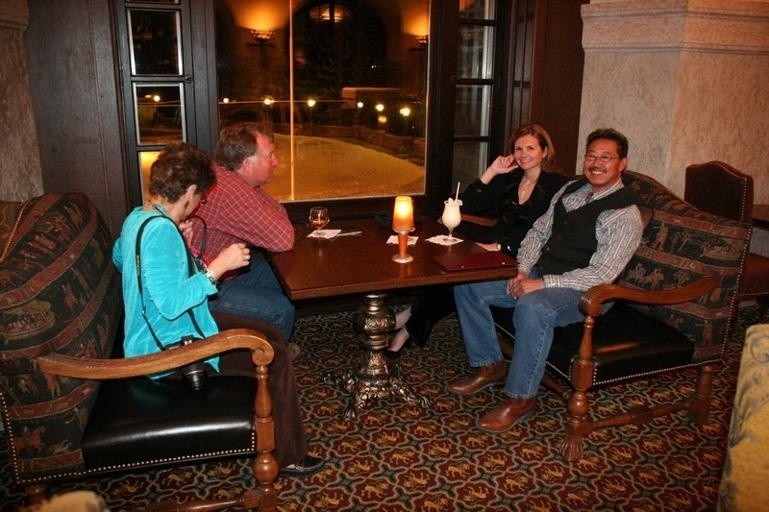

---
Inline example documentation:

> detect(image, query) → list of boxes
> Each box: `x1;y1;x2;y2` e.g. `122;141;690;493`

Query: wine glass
441;200;462;243
308;206;329;236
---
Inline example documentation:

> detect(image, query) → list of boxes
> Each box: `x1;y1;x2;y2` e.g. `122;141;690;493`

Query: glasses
584;153;622;162
198;188;208;206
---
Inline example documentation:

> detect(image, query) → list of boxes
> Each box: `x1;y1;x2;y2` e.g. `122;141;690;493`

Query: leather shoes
276;452;326;478
446;361;508;396
476;397;538;435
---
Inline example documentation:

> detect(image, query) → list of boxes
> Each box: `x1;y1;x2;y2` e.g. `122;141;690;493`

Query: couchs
476;168;754;462
2;189;291;511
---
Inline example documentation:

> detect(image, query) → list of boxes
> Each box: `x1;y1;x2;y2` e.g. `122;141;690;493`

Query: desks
260;213;520;423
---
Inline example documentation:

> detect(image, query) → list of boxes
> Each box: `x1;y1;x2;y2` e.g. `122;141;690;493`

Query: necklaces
519;178;535;202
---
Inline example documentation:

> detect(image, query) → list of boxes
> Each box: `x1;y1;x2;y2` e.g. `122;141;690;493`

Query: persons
110;142;325;479
379;123;569;361
445;126;644;435
181;115;300;364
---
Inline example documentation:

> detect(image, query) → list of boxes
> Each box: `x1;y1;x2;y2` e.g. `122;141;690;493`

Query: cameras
162;335;209;393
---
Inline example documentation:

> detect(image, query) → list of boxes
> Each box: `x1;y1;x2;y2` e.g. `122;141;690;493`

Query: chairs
680;159;769;331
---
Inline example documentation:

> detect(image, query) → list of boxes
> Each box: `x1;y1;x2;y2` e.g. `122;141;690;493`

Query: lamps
397;0;477;61
224;0;303;65
388;196;420;265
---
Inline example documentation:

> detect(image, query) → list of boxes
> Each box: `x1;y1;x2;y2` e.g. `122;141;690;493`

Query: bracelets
496;242;502;252
201;268;219;286
